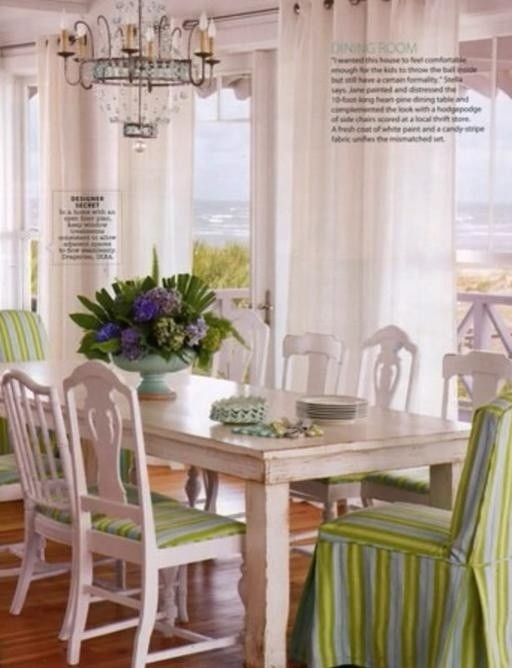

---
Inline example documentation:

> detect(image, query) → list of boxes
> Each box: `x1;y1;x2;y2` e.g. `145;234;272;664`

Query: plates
208;395;272;426
296;394;370;427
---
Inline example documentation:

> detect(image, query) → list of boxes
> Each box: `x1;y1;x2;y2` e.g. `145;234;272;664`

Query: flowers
67;245;255;369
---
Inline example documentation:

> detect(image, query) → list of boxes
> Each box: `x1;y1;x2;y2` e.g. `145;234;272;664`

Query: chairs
0;309;512;668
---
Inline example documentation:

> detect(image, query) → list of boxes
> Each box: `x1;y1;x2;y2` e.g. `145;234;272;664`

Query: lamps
57;0;221;152
